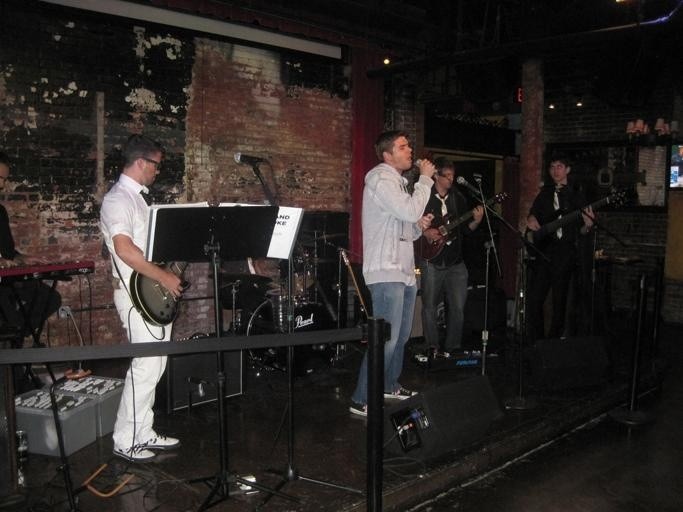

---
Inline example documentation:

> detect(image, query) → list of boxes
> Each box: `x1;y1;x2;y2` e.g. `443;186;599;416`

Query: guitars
413;192;507;260
130;261;191;327
524;190;626;257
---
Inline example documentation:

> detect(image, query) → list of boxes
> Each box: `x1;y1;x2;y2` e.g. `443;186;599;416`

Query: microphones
414;158;440;174
456;176;480;195
233;152;267;164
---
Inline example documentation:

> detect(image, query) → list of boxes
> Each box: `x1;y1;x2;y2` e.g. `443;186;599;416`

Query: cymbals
209;274;272;286
305;233;348;246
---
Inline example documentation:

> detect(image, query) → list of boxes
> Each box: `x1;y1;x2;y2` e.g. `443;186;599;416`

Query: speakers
155;349;242;413
352;375;505;471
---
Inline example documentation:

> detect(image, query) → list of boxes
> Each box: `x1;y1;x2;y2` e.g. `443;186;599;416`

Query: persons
100;134;183;464
525;159;595;348
420;164;483;357
350;134;435;417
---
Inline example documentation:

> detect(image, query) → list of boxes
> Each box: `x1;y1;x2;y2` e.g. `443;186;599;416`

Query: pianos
1;260;94;279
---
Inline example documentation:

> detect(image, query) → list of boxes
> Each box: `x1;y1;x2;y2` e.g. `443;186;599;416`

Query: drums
247;295;335;377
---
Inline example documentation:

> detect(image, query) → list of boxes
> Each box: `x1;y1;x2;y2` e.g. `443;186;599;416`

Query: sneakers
383;386;418;400
349;402;367;416
112;434;182;463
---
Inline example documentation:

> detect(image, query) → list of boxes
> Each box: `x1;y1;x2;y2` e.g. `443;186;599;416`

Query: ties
435;193;452;246
553;192;563;239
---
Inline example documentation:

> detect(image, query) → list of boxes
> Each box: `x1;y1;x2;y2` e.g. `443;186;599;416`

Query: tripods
184;235;372;512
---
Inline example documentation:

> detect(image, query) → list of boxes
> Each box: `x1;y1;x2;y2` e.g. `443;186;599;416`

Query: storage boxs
14;390;97;458
43;376;125;437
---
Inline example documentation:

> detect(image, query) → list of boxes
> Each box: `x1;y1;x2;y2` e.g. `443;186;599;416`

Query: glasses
141;156;162;170
